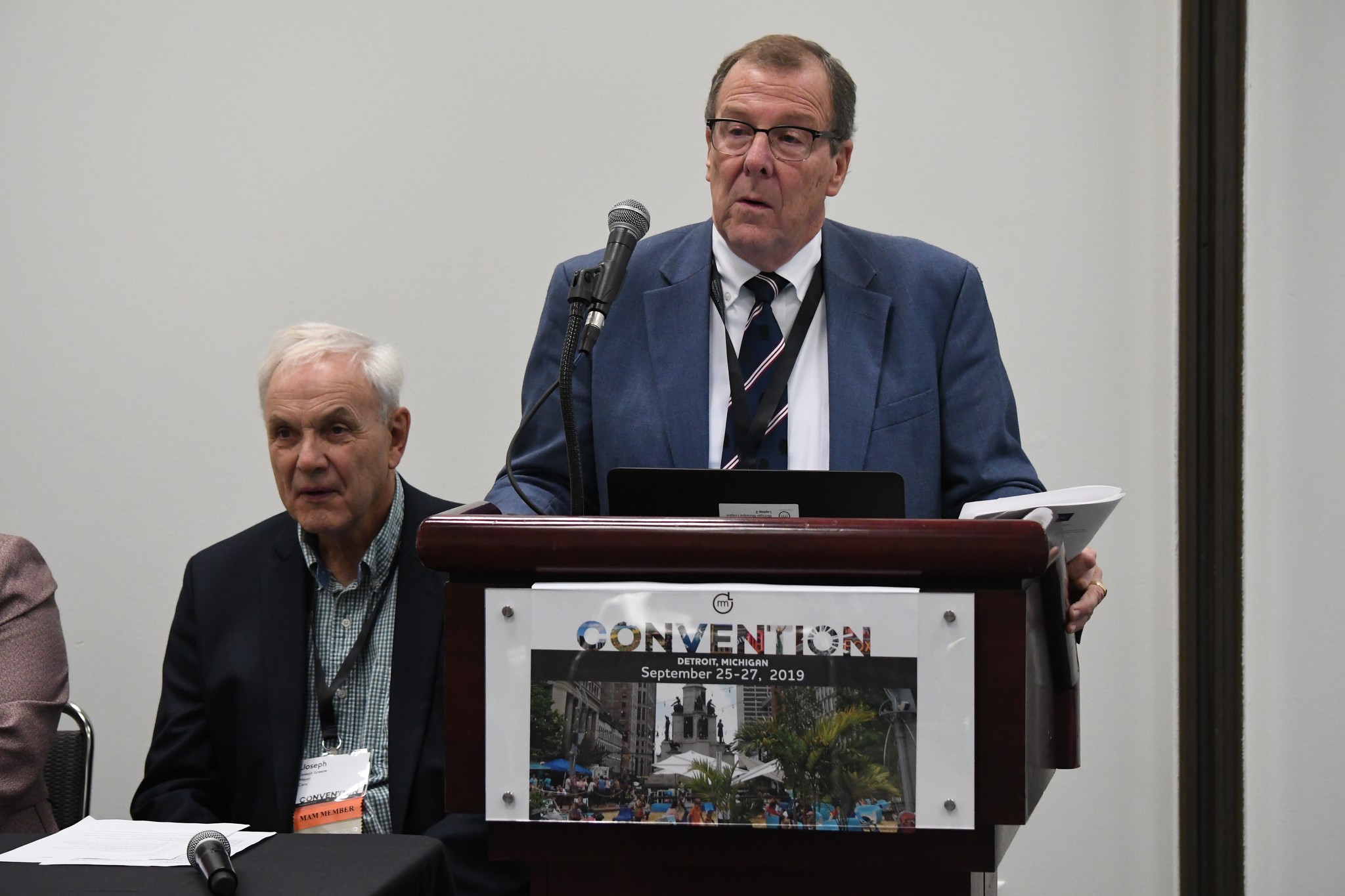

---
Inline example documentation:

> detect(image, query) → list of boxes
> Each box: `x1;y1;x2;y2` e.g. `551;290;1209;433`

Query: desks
1;834;452;896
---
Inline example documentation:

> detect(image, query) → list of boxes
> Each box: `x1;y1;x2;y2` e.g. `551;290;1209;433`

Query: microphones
186;829;238;896
577;198;651;356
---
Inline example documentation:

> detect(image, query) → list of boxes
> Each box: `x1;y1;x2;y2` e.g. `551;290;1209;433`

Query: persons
485;33;1108;644
128;319;468;834
0;534;70;836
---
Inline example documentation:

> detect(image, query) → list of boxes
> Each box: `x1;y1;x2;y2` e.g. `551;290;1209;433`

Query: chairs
43;700;95;831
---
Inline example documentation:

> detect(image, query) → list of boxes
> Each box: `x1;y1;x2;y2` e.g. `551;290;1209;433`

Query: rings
1090;581;1107;599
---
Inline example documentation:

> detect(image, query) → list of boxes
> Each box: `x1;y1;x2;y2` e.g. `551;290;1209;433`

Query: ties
719;271;791;470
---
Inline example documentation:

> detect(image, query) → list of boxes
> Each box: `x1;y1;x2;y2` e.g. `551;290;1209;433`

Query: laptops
606;466;907;519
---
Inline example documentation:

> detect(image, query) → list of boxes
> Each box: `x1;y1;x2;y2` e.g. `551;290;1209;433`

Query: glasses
706;117;836;161
